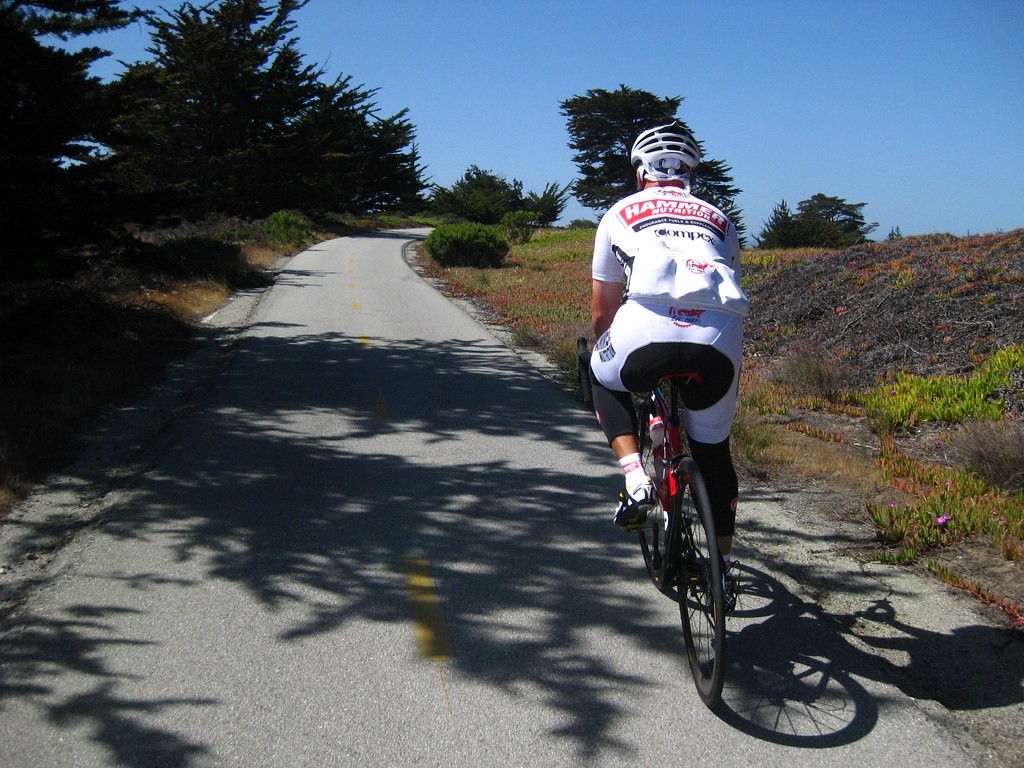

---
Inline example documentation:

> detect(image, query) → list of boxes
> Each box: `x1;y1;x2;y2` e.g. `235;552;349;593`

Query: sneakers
612;477;658;532
708;572;736;611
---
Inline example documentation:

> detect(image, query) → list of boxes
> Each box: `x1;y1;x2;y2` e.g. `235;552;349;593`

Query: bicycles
575;336;727;711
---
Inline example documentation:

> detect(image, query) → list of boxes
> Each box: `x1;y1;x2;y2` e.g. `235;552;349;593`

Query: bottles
649;415;664;450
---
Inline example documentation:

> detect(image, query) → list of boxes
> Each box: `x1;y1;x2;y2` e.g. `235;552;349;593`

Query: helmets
631;120;701;168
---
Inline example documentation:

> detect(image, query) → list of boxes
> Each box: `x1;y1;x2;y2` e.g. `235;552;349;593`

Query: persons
590;121;751;609
